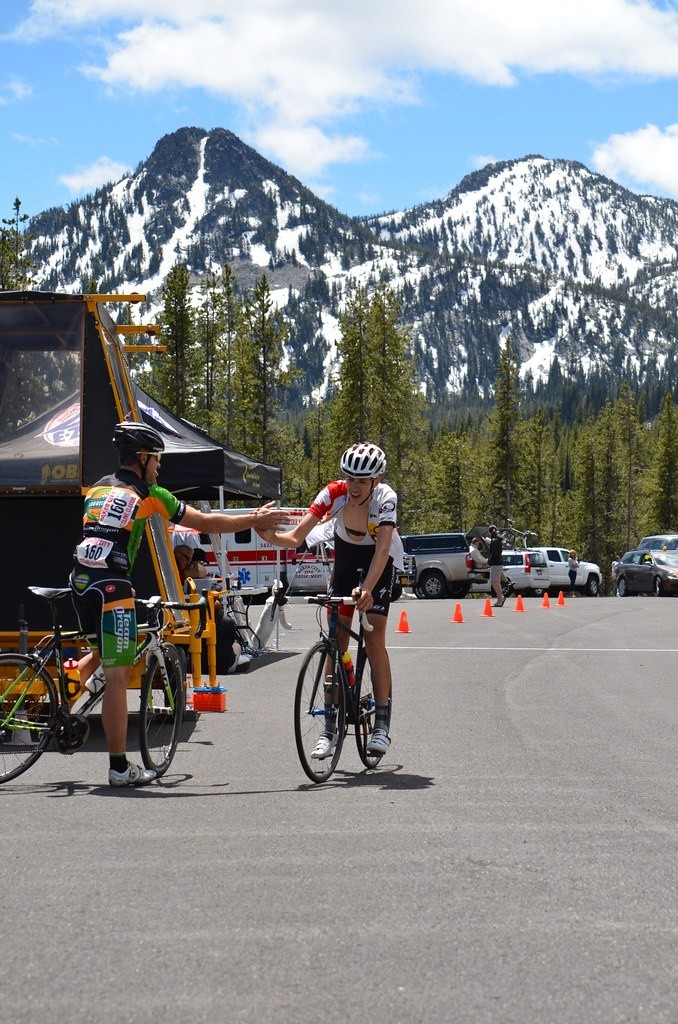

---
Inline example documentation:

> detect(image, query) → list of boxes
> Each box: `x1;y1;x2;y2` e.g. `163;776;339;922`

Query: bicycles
499;519;538;551
0;586;213;784
270;587;392;784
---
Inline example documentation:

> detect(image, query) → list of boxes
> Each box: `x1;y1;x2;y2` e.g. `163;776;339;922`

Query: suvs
391;552;418;603
615;549;678;597
527;547;602;598
398;523;491;599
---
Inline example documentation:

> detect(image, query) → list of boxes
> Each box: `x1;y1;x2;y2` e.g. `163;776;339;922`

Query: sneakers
366;727;392;753
109;761;157;787
309;728;340;759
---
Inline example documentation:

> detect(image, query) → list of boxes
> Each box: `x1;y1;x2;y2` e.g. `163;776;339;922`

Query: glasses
199;560;207;566
180;553;192;562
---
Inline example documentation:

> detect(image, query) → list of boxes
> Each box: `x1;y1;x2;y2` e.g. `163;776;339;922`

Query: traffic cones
480;598;496;617
555;591;567;606
449;603;466;623
395;611;411;633
513;595;528;612
539;593;551;608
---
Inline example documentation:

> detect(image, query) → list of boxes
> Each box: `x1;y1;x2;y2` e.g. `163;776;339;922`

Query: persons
568;550;580;598
69;421;292;785
612;556;620;597
469;525;516;607
249;442;404;759
173;545;250;676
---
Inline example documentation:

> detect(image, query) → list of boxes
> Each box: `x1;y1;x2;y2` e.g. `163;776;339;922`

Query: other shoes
507;581;516;587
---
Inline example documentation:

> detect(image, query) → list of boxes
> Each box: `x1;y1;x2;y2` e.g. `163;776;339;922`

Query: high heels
491;596;507;607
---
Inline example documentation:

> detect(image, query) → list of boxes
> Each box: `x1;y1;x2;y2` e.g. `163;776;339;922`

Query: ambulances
174;508;334;596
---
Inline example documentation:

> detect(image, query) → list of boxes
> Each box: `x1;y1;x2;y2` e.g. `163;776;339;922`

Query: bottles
340;650;357;686
213;572;242;591
85;664;107;693
64;657;81;701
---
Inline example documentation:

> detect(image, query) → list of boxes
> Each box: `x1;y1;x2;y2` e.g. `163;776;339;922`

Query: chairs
465;553;489;572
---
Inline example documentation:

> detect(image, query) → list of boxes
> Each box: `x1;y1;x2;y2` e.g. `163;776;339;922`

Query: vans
469;549;550;598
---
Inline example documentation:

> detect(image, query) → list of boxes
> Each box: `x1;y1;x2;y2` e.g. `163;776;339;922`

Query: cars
636;534;678;551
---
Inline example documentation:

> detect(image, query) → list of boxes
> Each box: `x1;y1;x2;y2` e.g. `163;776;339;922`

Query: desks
184;586;268;650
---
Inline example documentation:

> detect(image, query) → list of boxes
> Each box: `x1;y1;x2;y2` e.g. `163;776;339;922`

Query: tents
0;374;282;652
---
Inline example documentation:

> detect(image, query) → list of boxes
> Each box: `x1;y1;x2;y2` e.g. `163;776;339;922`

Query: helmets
340;441;386;478
112;420;166;455
